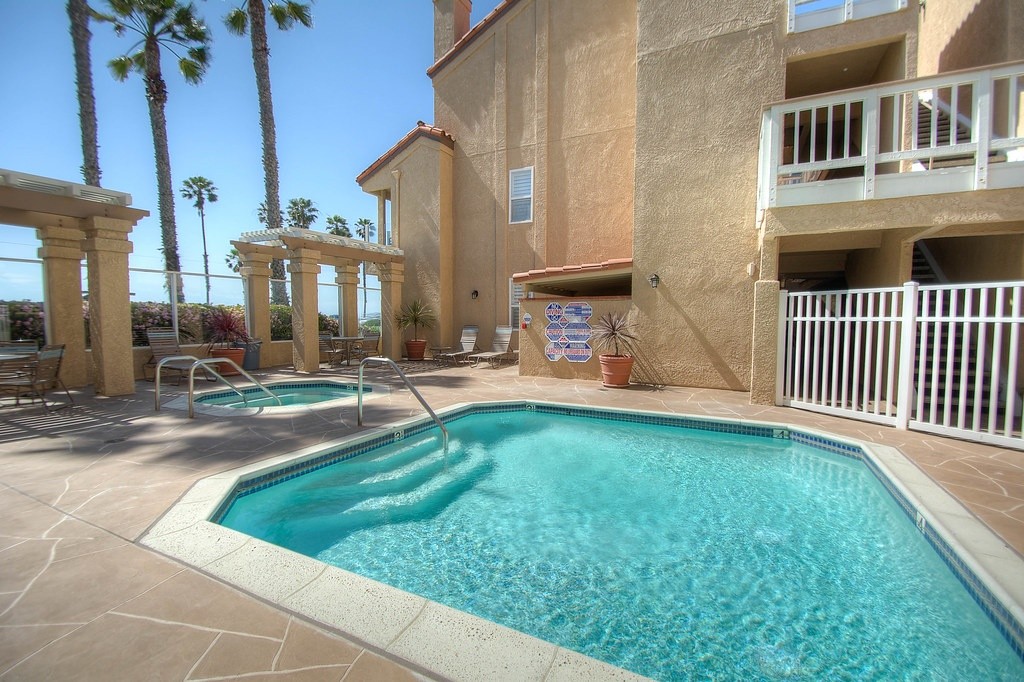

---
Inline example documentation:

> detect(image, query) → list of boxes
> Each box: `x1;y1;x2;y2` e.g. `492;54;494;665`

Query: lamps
472;290;479;299
747;263;756;276
650;274;660;288
778;275;788;289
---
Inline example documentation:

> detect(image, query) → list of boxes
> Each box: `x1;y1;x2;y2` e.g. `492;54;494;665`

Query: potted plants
393;298;437;361
589;311;642;388
197;306;257;376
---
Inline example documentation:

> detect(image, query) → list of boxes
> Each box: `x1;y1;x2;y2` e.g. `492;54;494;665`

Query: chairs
433;324;481;368
467;324;519;369
0;339;75;412
318;330;381;369
142;328;221;386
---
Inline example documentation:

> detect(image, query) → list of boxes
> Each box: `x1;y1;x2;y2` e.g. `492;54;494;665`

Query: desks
321;336;365;367
430;347;451;365
